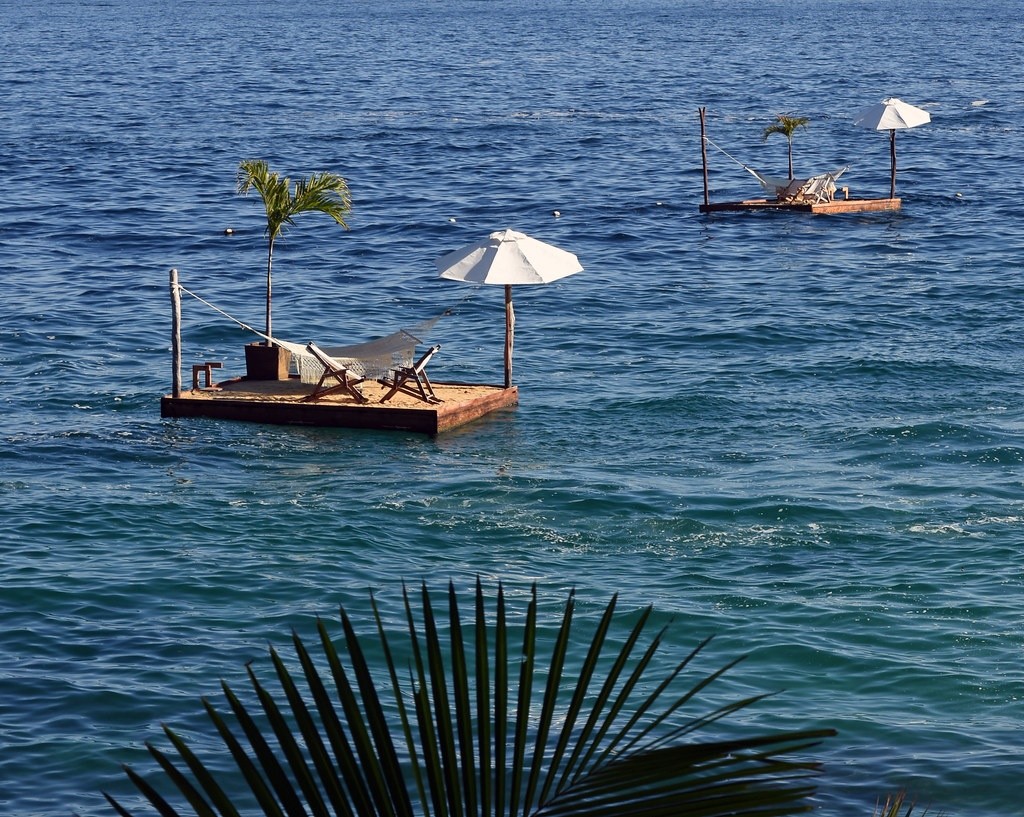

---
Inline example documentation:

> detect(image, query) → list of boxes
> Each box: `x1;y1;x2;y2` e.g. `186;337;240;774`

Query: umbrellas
852;98;931;197
433;229;584;389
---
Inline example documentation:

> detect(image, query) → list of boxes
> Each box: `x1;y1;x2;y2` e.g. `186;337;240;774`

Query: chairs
376;344;446;405
777;178;809;204
298;340;369;404
801;177;831;204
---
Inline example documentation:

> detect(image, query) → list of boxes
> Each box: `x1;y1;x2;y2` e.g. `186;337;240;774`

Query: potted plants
236;157;354;381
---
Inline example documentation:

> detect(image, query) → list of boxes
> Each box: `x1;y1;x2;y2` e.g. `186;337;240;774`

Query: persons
827;186;850;201
